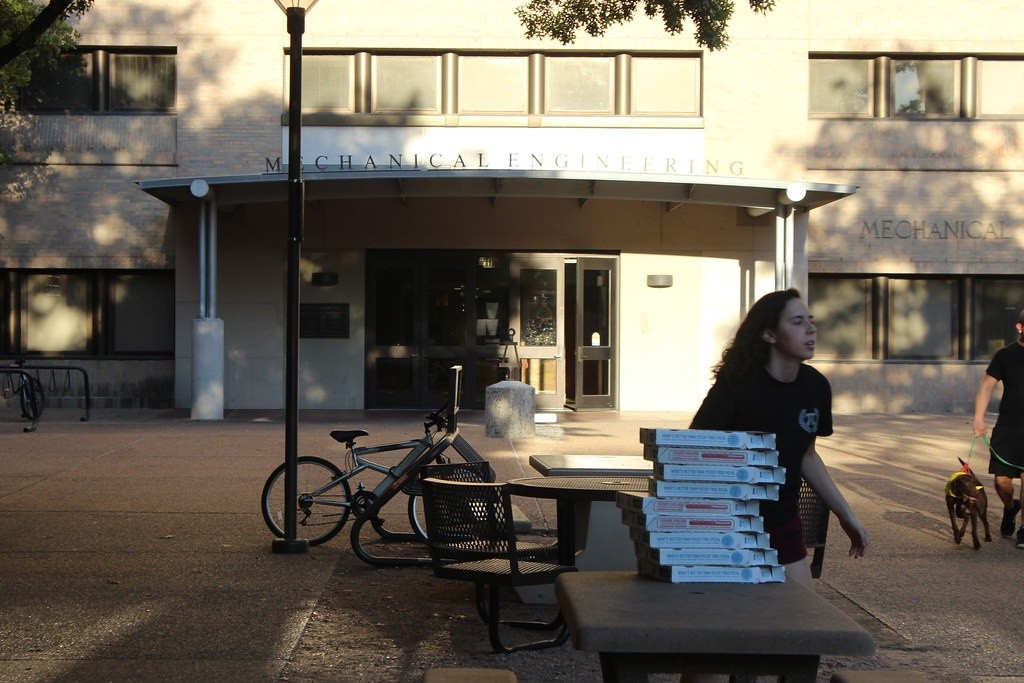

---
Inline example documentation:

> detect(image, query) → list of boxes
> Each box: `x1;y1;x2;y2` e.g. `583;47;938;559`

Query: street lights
272;0;319;554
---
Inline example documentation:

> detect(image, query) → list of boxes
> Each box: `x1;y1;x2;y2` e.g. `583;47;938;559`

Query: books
616;428;790;583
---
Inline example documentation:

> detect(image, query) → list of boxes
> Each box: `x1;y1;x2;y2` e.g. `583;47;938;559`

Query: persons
972;306;1024;547
680;289;868;683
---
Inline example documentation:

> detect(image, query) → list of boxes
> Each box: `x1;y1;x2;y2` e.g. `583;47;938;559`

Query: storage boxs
637;559;785;583
648;475;780;502
634;543;781;567
643;444;779;468
629;528;770;549
653;462;786;485
617;491;759;517
639;428;777;451
621;508;764;533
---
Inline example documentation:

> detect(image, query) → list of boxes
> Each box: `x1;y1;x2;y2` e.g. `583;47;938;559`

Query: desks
507;475;653;572
556;570;874;683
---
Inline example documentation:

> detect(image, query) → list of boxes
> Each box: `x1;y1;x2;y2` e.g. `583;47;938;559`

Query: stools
422;669;517;683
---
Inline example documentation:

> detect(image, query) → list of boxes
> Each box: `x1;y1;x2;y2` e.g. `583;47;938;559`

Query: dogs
945;457;992;551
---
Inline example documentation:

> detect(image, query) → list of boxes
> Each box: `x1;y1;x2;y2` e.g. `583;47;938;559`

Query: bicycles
14;350;45;422
260;400;496;558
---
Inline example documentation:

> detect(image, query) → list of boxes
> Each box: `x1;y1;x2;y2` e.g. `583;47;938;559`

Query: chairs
420;461;557;564
420;478;568;652
